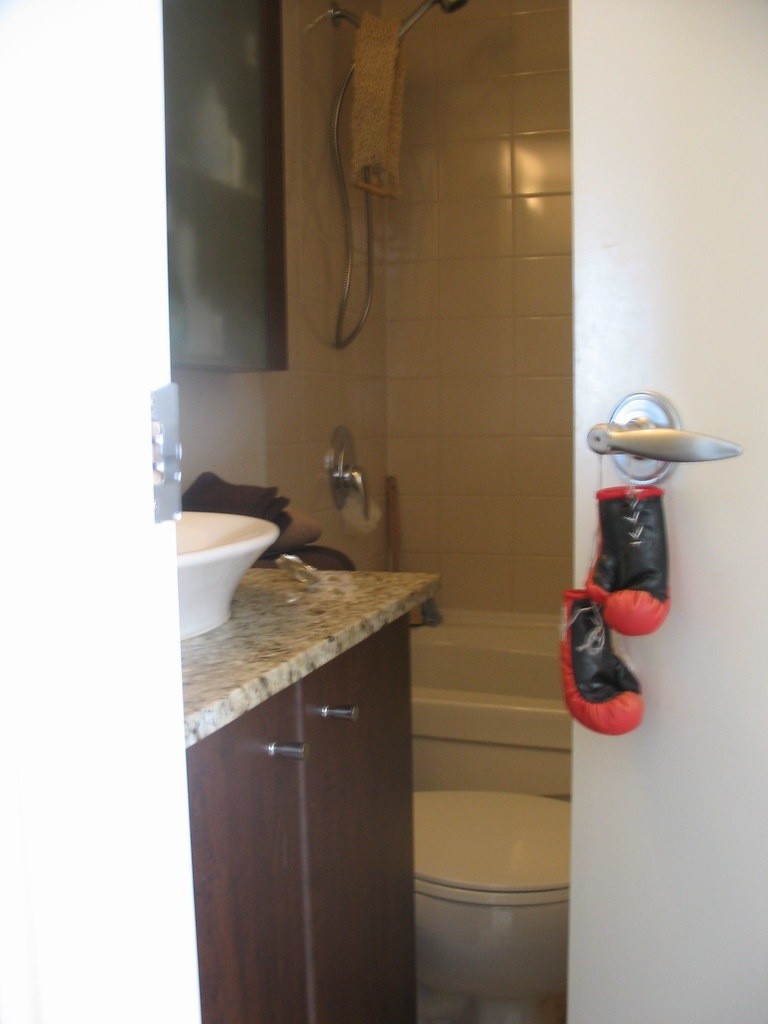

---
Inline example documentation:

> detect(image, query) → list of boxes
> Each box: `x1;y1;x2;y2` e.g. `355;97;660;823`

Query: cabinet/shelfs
186;612;416;1024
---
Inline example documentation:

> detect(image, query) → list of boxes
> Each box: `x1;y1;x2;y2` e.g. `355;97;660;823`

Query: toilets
411;787;569;1017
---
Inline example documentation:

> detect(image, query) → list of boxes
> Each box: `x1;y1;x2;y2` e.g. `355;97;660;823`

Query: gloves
584;483;671;637
558;585;647;738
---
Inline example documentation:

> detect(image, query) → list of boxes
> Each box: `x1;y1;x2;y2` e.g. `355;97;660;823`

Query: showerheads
397;2;474;40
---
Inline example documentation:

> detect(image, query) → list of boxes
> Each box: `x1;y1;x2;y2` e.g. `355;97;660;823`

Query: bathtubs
410;607;572;799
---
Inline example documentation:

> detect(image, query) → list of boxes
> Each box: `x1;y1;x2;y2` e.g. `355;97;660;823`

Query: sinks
178;511;282;641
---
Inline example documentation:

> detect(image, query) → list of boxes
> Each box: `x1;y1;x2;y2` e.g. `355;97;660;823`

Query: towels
348;11;411;201
